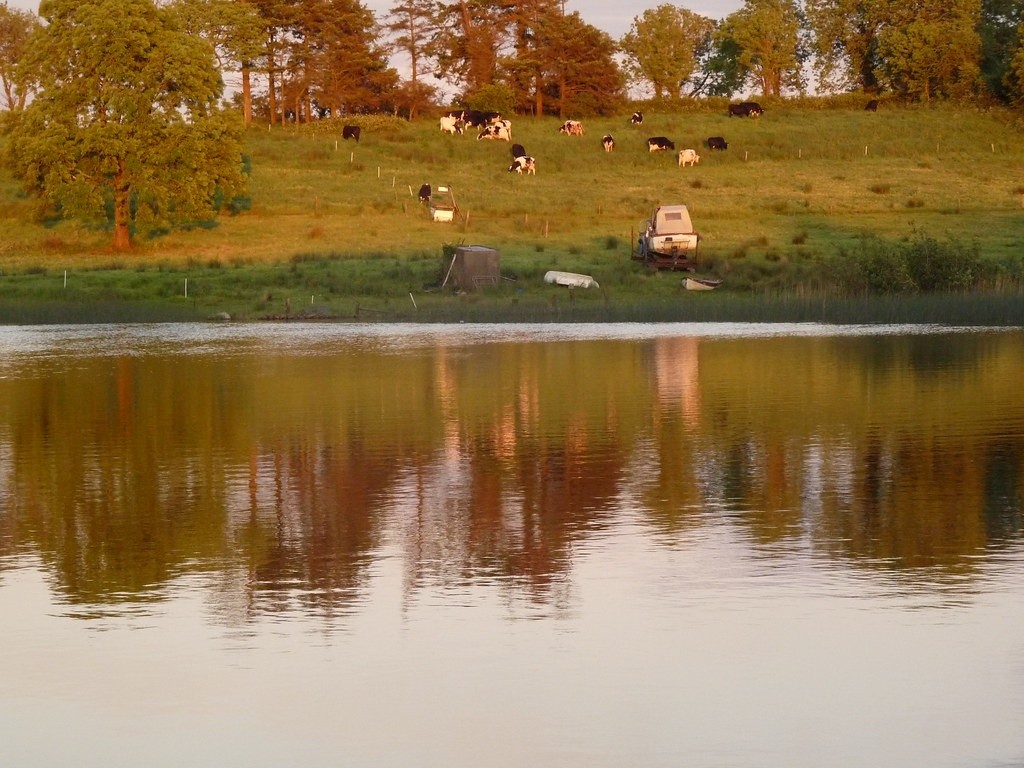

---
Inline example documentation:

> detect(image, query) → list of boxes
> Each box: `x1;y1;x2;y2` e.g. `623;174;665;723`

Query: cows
631;111;644;125
506;143;536;176
601;133;615;153
678;148;701;167
419;182;431;205
864;99;881;112
558;119;585;137
728;102;765;119
708;137;729;152
439;110;512;142
342;124;361;144
648;136;675;153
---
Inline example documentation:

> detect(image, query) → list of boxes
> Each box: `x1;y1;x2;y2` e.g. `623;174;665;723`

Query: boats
646;204;699;256
